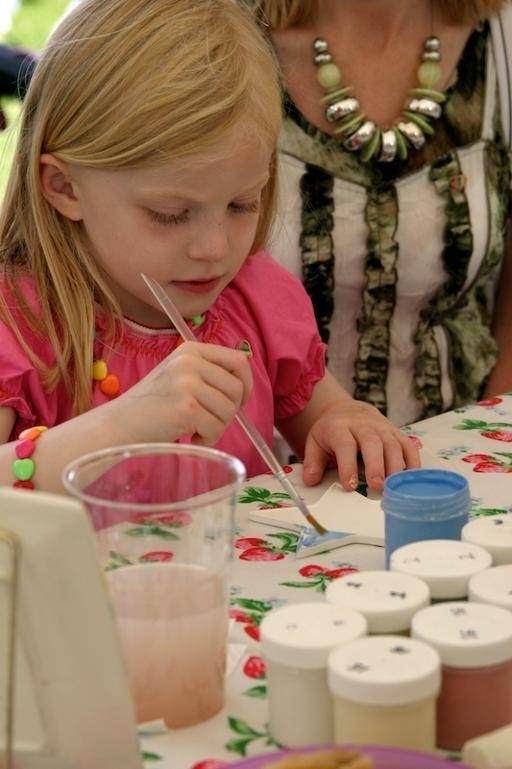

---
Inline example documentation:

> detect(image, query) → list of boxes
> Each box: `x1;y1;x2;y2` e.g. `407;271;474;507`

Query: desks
87;391;512;769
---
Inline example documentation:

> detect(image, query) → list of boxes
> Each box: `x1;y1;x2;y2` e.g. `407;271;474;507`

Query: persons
0;1;422;531
217;0;512;465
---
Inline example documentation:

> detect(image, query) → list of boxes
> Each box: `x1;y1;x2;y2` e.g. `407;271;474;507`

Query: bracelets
12;424;47;490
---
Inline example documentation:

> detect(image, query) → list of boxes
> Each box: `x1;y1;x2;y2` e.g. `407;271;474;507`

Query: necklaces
309;37;448;164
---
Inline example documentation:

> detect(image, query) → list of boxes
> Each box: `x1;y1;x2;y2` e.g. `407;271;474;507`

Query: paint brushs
139;270;329;538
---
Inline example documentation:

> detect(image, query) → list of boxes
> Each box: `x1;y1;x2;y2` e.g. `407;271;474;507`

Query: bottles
260;601;366;749
411;603;512;749
459;513;512;558
327;569;429;634
468;562;512;610
387;539;493;601
327;634;436;752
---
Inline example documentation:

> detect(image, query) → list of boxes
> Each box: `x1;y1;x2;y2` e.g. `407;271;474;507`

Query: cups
380;468;473;569
61;444;247;731
